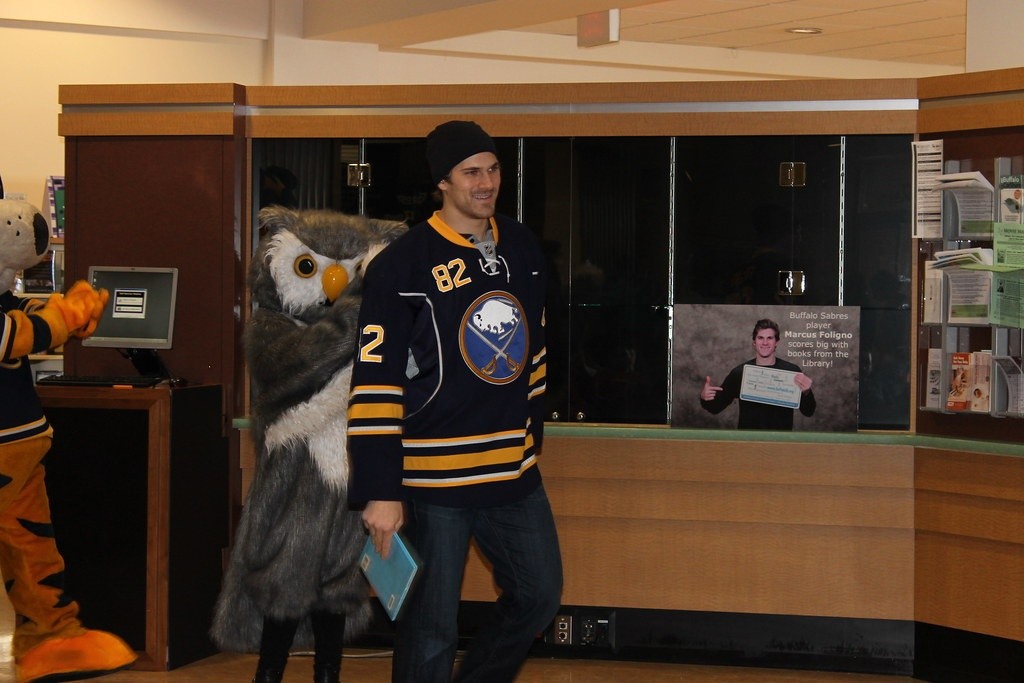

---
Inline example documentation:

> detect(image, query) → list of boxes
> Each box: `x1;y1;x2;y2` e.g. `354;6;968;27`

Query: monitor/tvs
81;266;178;376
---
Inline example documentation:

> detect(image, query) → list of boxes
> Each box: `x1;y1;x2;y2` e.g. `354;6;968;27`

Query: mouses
169;377;187;387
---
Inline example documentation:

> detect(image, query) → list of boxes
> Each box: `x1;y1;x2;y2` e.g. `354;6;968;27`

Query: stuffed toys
0;176;136;683
209;208;410;683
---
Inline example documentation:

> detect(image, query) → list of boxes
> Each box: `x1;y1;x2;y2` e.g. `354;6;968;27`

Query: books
358;531;418;621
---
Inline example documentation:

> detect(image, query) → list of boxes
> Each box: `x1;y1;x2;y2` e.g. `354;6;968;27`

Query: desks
32;382;229;672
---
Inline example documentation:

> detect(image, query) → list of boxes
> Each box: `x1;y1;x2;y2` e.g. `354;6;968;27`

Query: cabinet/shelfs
13;237;64;360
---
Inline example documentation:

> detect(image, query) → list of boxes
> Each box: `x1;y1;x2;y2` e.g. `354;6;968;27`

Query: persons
346;121;564;683
700;319;816;432
1005;190;1023;213
972;388;990;407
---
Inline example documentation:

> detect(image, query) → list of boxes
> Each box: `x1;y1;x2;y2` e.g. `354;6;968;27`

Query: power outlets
553;615;572;645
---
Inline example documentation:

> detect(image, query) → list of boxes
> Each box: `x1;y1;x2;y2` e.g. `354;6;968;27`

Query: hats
425;120;496;186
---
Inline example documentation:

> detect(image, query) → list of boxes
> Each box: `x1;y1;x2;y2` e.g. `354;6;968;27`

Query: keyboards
36;375;156;388
